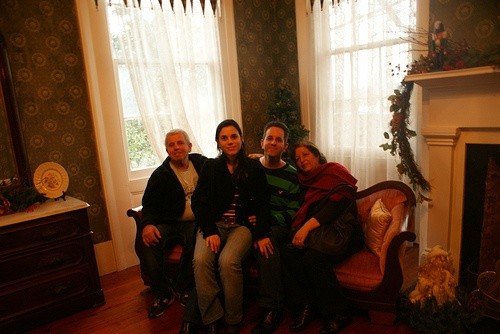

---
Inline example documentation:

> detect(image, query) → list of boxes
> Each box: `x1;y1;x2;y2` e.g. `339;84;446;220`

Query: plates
33;162;70;198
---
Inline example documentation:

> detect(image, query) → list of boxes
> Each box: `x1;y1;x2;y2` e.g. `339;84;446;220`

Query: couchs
127;181;419;322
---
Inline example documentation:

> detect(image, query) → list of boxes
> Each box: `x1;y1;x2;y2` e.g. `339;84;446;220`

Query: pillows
364;195;394;259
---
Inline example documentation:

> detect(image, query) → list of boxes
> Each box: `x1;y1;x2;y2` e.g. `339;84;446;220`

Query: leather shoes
290;304;311;332
320;313;352;334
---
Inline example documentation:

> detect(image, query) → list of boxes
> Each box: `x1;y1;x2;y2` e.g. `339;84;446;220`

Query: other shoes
251;309;285;334
207;321;218;334
225;324;241;334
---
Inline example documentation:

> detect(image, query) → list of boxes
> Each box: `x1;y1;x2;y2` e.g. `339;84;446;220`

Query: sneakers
149;290;175;318
178;294;188;307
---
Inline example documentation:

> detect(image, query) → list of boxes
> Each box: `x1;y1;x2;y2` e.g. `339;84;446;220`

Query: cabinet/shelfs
0;197;108;334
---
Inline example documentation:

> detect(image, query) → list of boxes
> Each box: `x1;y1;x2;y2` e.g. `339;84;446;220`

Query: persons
249;142;360;334
190;118;274;334
247;121;299;334
135;129;215;320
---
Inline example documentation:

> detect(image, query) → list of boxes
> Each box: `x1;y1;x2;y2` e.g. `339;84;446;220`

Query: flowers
379;44;476;203
